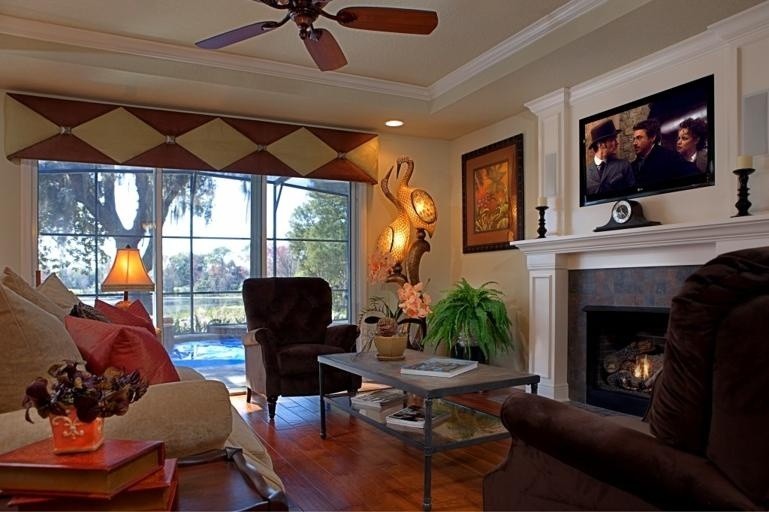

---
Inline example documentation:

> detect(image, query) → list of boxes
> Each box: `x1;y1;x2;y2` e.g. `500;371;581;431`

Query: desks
0;444;287;512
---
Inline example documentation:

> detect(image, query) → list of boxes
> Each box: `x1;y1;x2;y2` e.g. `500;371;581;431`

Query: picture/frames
461;133;525;254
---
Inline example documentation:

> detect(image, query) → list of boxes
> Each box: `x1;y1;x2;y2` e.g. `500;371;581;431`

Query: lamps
367;153;438;351
100;245;155;309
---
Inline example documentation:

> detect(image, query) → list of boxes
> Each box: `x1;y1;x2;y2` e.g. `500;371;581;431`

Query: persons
628;119;703;190
586;119;639;202
675;117;707;172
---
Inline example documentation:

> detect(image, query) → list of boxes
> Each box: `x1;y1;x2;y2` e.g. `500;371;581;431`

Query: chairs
480;245;769;512
238;276;363;420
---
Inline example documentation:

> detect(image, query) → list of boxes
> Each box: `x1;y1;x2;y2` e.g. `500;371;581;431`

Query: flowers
23;359;149;424
335;282;433;357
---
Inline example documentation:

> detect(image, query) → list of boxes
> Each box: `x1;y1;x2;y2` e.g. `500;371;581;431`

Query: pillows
0;266;181;413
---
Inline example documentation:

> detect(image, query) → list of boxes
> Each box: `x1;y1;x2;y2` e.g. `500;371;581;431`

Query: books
0;435;165;502
399;355;478;378
386;404;452;428
350;388;408;409
7;456;178;512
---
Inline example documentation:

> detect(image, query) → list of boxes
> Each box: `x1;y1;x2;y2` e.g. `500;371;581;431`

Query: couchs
0;266;285;493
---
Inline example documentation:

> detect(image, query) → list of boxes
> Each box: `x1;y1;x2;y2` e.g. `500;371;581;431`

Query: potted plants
420;278;516;365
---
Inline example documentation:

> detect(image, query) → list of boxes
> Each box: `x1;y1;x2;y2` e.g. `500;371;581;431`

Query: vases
372;334;408;361
47;405;105;454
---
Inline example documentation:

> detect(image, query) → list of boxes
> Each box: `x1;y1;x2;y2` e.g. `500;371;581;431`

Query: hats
588;118;623;150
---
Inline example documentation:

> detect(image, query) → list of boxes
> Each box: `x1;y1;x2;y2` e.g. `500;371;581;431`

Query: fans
195;0;439;70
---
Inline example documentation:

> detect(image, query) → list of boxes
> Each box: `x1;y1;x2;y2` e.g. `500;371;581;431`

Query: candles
537;195;548;207
736;155;754;168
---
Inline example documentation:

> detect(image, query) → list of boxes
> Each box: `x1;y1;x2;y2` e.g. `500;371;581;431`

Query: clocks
594;199;662;232
